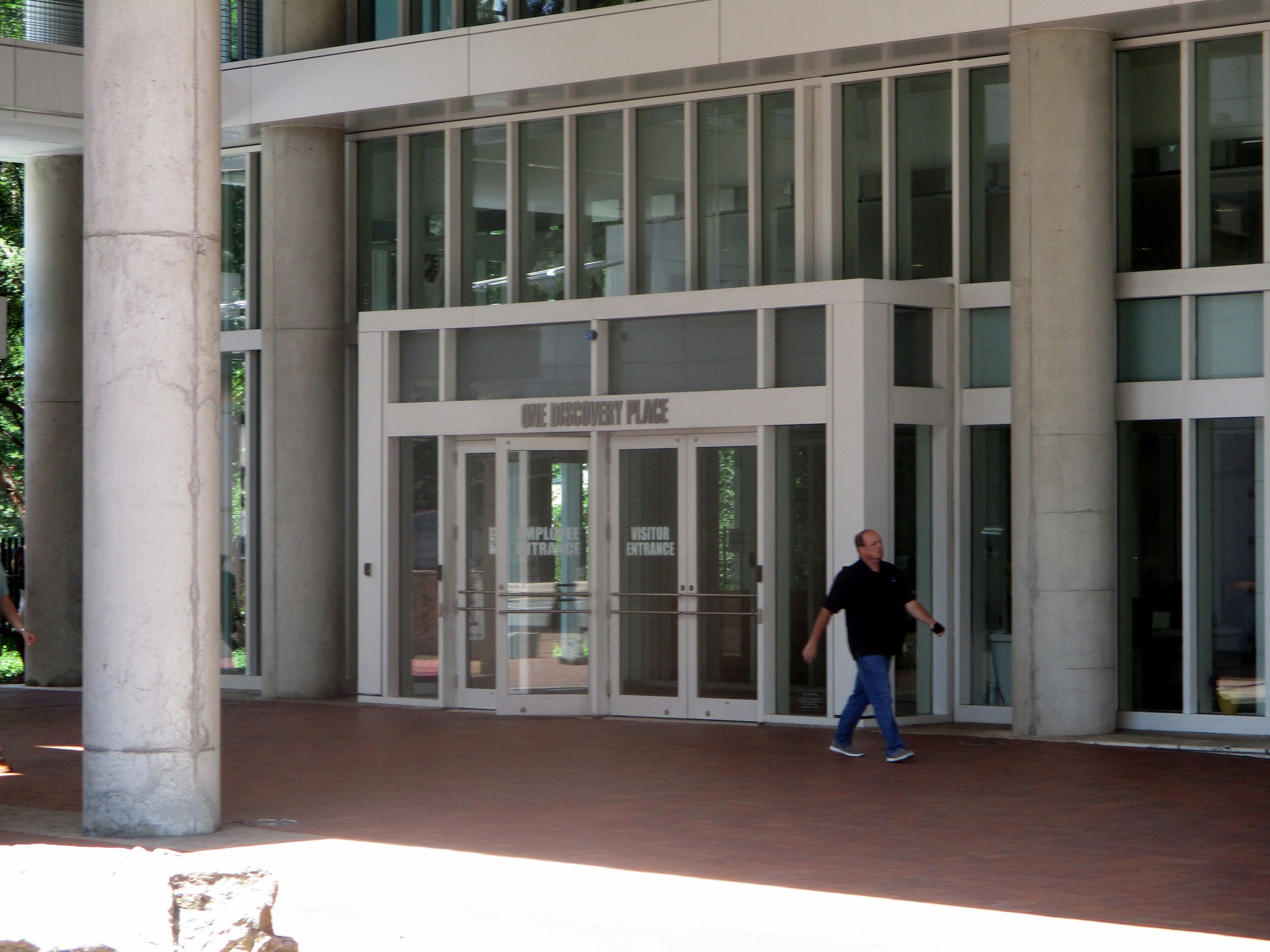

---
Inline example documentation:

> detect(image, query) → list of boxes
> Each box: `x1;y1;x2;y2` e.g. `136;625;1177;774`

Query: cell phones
931;622;945;634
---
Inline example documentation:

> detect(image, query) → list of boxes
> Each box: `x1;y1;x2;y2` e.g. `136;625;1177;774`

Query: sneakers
828;739;866;758
0;756;15;774
886;749;914;765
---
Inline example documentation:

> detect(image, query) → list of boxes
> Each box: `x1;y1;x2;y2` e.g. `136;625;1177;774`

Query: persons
0;562;36;773
801;528;947;762
219;553;243;669
